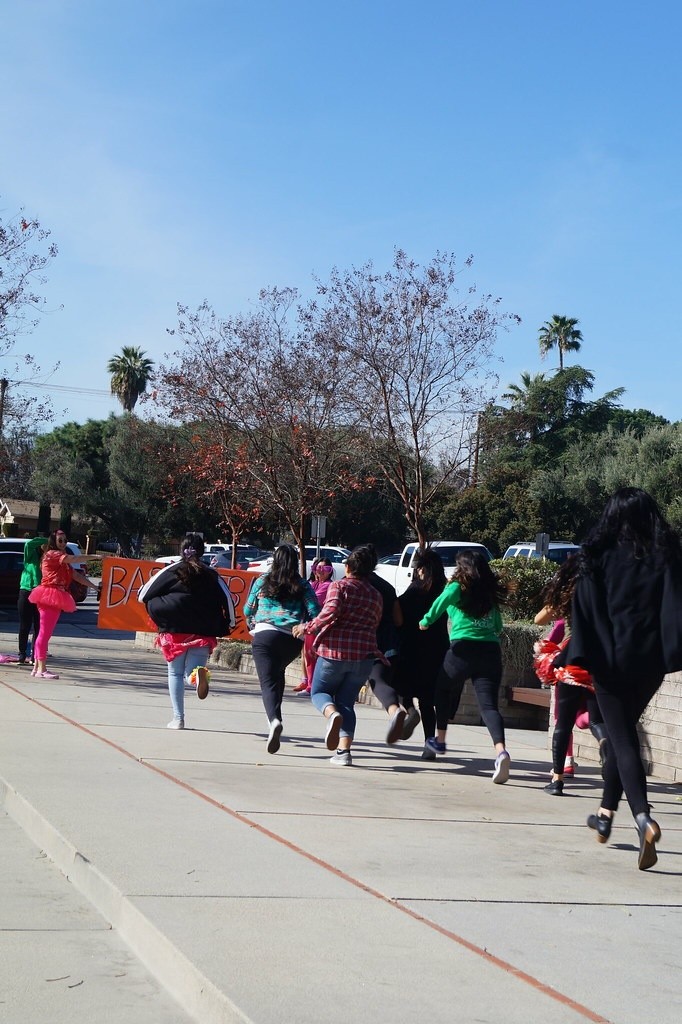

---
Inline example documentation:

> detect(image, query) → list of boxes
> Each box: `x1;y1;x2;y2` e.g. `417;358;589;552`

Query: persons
244;544;323;755
28;530;103;679
419;551;510;785
292;543;406;765
18;537;49;666
533;553;607;795
398;550;450;761
554;487;682;871
137;533;236;730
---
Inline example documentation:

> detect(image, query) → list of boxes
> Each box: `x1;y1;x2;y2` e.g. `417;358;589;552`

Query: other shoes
196;667;209;700
421;749;436;759
166;719;184;729
292;682;307;691
329;752;352;765
398;710;420;740
325;711;343;751
19;652;27;662
46;650;52;657
384;708;406;744
267;718;284;754
543;780;564;794
296;690;311;696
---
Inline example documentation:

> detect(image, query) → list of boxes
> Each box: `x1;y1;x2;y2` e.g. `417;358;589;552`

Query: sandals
31;669;59;679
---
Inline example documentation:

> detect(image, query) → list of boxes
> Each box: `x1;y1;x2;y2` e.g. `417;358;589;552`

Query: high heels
586;812;614;843
637;818;661;870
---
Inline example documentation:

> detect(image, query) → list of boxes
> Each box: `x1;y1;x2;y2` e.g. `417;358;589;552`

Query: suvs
0;538;88;612
500;540;583;571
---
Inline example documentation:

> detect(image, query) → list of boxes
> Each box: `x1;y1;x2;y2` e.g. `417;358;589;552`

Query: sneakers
426;736;446;754
549;766;575;777
598;738;607;766
492;750;510;784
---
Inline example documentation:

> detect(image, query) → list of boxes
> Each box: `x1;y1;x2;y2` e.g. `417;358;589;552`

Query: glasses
310;564;333;574
55;539;68;544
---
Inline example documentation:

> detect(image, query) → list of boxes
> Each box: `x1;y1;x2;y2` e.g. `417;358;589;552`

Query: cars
153;539;496;600
96;536;145;555
245;544;355;582
